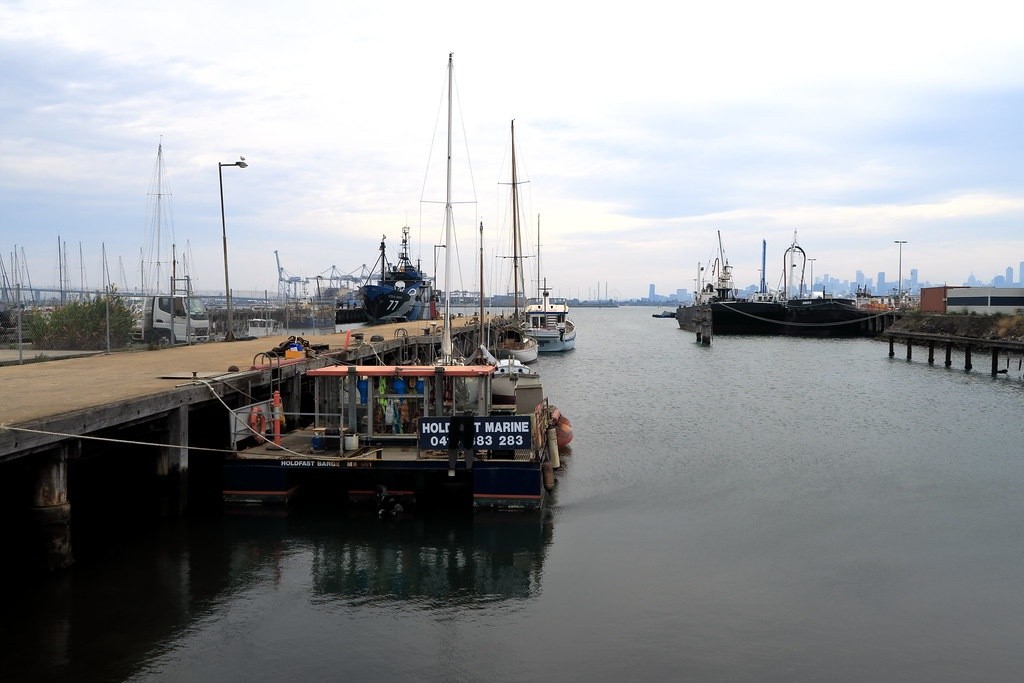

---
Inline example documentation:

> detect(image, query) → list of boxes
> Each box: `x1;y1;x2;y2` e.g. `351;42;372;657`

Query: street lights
218;160;248;341
894;239;909;303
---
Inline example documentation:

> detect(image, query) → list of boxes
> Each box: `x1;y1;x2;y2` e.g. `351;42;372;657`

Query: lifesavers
534;405;542;448
250;407;266;443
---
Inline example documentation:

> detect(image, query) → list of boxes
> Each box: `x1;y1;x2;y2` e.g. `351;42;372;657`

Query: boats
359;231;443;328
518;277;576;351
486;120;540;366
245;318;285;339
673;226;866;338
213;51;571;527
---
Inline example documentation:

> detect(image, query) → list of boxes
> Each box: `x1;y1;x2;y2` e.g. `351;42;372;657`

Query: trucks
128;295;211;349
